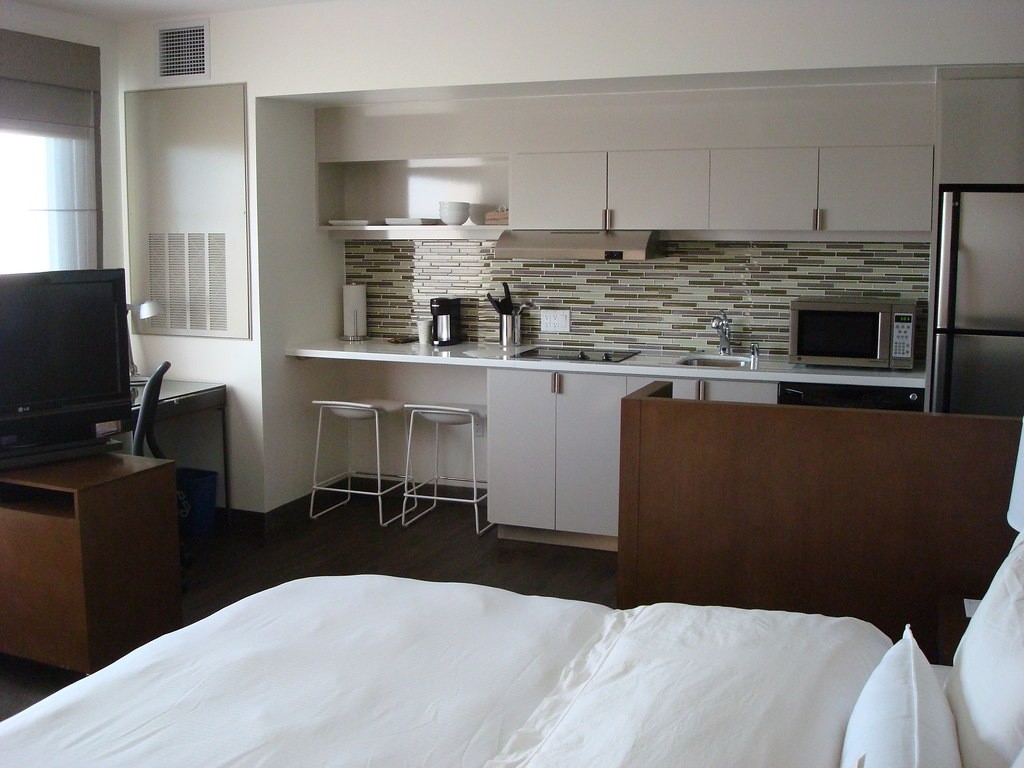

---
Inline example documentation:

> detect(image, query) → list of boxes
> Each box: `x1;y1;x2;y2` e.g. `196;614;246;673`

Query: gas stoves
510;346;641;362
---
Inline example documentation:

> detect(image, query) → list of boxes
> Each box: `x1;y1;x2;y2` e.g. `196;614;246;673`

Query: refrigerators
924;183;1024;417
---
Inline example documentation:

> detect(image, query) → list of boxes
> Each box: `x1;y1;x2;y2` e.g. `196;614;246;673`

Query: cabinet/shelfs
484;365;779;553
506;147;935;244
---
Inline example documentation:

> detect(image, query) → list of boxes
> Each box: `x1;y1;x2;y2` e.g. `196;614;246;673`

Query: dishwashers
777;381;925;413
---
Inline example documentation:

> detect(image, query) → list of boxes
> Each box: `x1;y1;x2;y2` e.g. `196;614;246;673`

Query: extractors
494;230;659;261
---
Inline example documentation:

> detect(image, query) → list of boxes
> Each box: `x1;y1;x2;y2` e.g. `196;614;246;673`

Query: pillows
944;539;1023;768
837;622;964;768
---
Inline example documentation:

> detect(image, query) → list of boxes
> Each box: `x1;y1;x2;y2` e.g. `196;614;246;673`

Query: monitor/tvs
0;268;133;455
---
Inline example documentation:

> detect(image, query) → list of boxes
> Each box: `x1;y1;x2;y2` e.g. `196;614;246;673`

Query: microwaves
788;295;917;369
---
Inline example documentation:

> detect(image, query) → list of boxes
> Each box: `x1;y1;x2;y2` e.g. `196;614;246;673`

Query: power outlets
540;309;571;333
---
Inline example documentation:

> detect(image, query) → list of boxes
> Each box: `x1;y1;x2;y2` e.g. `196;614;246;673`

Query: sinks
677;353;751;368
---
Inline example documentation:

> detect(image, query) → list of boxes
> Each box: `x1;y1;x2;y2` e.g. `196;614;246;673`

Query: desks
0;378;231;523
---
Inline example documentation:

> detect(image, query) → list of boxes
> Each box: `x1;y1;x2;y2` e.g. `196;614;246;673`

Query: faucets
711;309;735;356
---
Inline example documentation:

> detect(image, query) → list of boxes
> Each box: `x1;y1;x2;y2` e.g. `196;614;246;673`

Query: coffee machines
430;298;459;346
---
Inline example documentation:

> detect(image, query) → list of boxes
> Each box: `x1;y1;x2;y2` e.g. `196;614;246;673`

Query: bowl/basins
470;204;499;225
439;201;470;225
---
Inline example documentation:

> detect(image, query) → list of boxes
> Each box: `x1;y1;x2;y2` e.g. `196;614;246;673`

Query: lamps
126;300;163;376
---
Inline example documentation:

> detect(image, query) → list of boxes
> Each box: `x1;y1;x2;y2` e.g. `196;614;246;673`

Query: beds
0;574;947;768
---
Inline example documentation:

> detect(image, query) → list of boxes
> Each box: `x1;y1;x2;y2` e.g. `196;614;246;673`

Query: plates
328;220;385;225
385;217;436;224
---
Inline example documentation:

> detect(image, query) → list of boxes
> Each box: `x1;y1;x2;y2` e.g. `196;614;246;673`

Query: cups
500;314;522;346
417;321;433;342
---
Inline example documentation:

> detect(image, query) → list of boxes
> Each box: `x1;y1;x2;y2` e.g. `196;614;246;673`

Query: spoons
516;302;532;315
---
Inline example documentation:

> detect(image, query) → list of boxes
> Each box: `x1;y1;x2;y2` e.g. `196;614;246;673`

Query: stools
401;404;496;537
307;400;418;529
